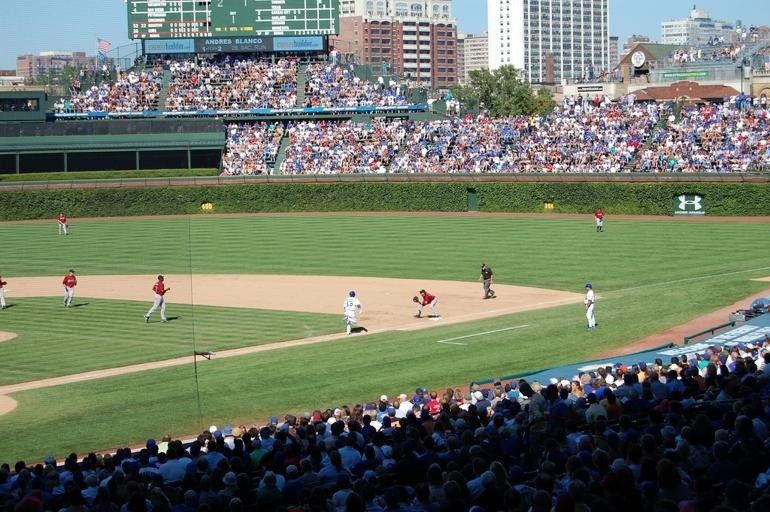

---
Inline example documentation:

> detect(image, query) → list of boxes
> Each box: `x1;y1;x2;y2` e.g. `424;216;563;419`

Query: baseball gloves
413;296;418;301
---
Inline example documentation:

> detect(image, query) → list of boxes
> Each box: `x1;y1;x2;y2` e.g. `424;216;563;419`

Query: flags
97;38;111;66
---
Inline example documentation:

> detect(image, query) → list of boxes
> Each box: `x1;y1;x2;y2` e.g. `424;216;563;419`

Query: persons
57;210;69;238
478;260;496;299
583;282;597;330
0;277;8;311
341;290;364;338
54;45;770;179
143;275;172;325
704;23;761;46
62;269;78;309
593;203;605;232
1;328;770;512
412;287;440;321
